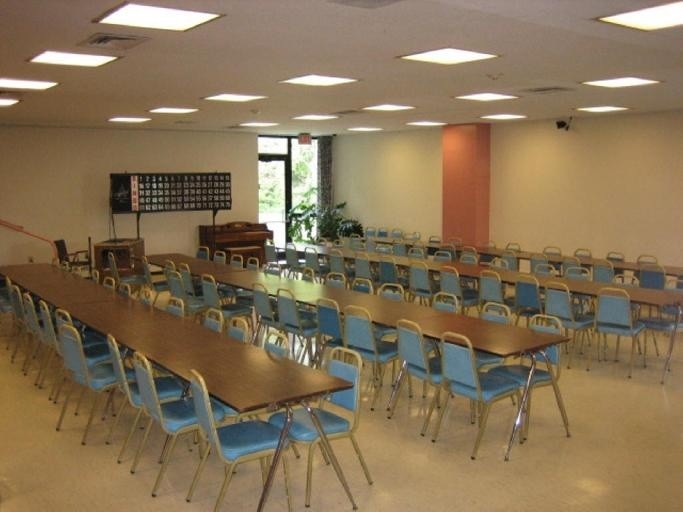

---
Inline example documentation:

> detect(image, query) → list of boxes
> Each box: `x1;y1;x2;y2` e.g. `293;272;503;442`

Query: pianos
199;221;273;266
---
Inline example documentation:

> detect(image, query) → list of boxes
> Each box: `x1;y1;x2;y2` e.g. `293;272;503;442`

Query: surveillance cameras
556;119;567;130
